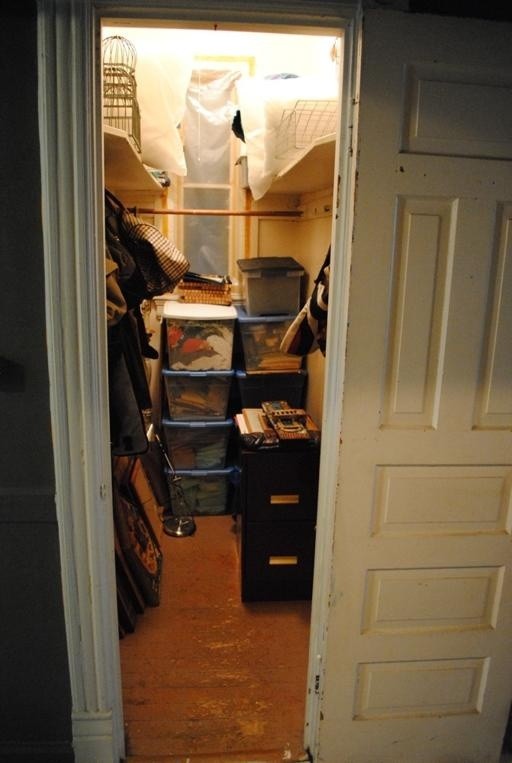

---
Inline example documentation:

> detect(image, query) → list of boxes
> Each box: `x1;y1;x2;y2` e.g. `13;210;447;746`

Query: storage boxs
163;408;233;470
164;466;234;514
163;300;238;371
236;256;305;317
237;306;304;370
161;368;234;422
236;370;307;408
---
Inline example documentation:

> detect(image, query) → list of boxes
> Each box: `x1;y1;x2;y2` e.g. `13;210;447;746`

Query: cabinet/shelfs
234;441;320;602
